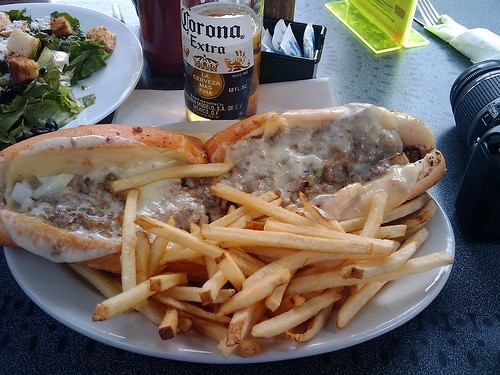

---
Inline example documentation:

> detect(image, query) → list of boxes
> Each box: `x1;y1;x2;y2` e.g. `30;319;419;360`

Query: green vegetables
0;7;112;145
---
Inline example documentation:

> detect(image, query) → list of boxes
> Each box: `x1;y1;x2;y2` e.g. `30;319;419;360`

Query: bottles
133;0;186;91
180;0;263;121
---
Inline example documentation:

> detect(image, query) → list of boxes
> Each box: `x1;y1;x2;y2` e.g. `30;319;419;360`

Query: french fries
66;161;456;359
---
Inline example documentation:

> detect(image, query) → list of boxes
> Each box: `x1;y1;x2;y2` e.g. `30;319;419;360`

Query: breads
0;99;446;270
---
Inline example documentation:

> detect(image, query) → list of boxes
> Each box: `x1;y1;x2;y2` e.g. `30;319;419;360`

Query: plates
3;117;459;363
1;2;143;141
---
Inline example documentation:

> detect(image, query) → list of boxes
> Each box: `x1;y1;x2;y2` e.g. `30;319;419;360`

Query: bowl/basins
260;13;325;82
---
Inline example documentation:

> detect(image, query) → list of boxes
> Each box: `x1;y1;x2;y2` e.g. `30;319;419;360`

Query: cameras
450;60;500;238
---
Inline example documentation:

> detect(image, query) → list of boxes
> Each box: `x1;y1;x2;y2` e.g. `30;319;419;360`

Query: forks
418;0;443;26
